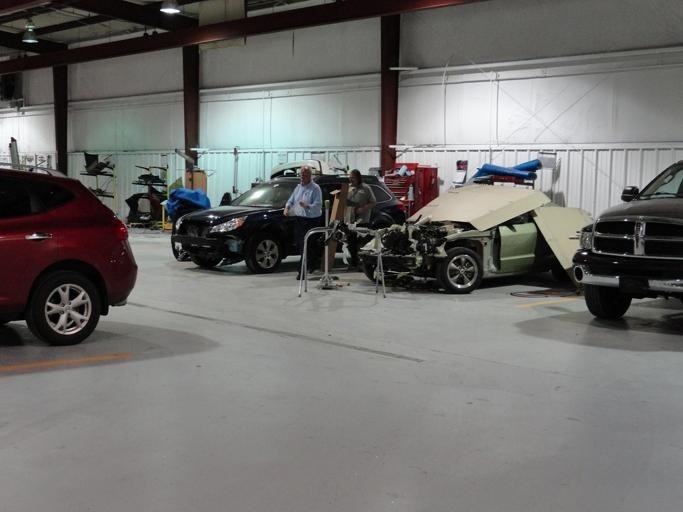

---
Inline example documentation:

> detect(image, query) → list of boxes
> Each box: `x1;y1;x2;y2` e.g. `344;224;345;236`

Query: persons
341;169;377;273
282;164;322;280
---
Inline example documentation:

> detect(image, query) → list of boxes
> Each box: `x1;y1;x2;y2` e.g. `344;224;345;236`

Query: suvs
0;162;137;346
170;172;406;274
572;160;683;320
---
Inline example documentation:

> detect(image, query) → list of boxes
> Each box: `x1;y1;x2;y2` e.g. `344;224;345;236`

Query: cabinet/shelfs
385;164;438;218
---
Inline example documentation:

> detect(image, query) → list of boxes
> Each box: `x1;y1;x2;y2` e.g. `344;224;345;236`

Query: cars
356;183;571;294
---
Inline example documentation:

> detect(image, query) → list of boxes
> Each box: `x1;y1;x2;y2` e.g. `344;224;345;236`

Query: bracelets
307;204;309;210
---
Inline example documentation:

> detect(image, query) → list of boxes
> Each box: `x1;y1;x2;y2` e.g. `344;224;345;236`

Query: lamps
160;0;181;14
22;29;38;43
